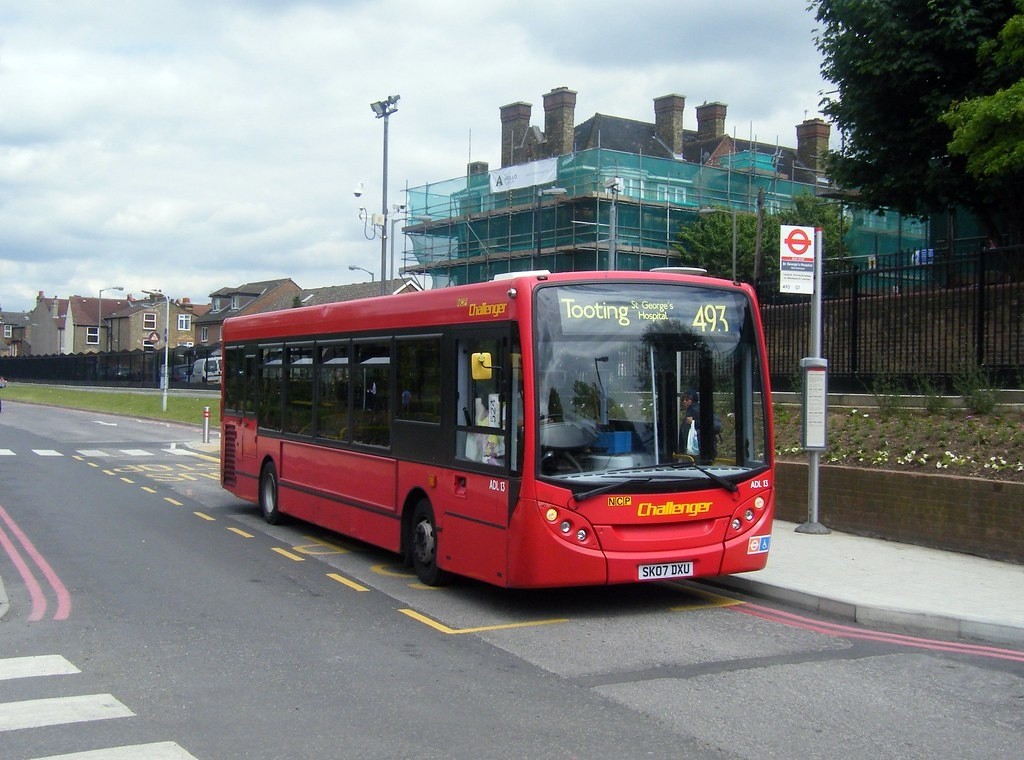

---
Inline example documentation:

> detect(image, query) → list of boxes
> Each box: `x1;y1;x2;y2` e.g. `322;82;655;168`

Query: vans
191;356;223;385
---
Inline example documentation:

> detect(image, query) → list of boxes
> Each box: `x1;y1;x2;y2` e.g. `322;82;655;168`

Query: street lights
142;289;169;412
390;216;433;295
96;287;125;352
369;95;402;296
349;266;375;283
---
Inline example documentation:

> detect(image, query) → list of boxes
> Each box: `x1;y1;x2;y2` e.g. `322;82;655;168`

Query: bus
220;266;777;590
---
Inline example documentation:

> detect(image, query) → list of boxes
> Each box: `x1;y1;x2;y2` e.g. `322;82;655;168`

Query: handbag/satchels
687;420;700;456
679;420;691;454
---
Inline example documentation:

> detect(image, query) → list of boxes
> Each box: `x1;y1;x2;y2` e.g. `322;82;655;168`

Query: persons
401;388;412;416
679;389;718;461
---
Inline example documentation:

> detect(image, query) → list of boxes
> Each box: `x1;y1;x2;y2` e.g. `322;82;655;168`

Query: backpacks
713;412;721;434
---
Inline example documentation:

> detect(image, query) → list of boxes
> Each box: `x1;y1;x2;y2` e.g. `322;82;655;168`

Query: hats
684;388;698;401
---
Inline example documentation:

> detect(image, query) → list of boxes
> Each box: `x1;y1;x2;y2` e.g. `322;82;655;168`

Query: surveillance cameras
605;177;620;188
353;189;362;197
393;203;405;210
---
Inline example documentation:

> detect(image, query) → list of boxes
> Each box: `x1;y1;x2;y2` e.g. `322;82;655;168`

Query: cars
174;363;193;382
